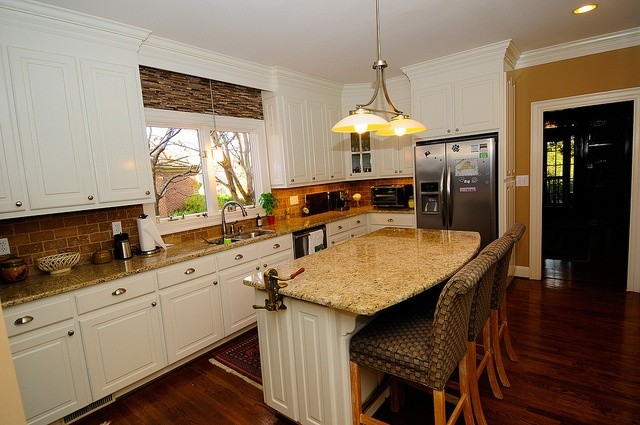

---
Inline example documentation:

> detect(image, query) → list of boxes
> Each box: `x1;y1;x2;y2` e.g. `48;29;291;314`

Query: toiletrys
256;212;262;226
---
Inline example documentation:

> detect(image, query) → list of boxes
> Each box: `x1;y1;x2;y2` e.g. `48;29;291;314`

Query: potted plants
259;193;281;225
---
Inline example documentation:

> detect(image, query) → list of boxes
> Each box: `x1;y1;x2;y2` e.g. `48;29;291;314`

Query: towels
301;229;324;255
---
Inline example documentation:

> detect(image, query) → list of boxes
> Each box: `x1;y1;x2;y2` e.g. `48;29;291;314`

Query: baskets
35;251;80;274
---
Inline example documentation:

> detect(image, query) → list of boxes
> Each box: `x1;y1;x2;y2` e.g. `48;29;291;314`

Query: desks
243;227;481;425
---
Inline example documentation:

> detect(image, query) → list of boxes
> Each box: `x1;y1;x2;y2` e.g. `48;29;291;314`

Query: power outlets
0;239;11;255
289;196;299;205
111;221;122;236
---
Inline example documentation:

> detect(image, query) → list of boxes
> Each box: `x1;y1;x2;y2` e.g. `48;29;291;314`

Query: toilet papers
135;218;169;253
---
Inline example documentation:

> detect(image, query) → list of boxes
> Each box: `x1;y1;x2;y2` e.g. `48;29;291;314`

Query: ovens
292;224;328;259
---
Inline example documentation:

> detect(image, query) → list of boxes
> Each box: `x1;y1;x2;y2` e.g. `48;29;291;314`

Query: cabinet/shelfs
307;100;329;186
326;219;347;249
261;96;310;189
375;97;414;180
324;103;345;184
74;269;170;403
77;55;157;208
1;291;92;425
155;253;224;367
348;212;368;239
342;105;375;182
0;47;30;218
8;45;99;216
217;234;293;340
367;211;416;233
411;73;516;142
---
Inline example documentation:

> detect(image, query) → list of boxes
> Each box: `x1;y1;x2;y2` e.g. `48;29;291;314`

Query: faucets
221;200;247;234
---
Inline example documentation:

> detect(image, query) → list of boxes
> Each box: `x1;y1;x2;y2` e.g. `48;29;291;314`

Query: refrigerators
414;131;500;251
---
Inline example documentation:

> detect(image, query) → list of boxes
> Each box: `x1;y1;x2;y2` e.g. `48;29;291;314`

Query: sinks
208;238;239;245
229;231;270;239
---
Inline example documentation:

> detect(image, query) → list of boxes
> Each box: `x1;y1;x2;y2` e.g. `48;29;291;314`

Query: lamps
331;0;426;138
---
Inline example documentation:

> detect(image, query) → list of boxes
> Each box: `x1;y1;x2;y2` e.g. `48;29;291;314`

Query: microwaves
370;183;413;208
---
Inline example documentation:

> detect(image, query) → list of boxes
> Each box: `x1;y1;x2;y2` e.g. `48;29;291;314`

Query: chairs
467;236;518;425
349;255;495;425
491;224;527;390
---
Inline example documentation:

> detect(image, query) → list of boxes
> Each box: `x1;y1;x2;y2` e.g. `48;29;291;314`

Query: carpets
209;326;262;391
